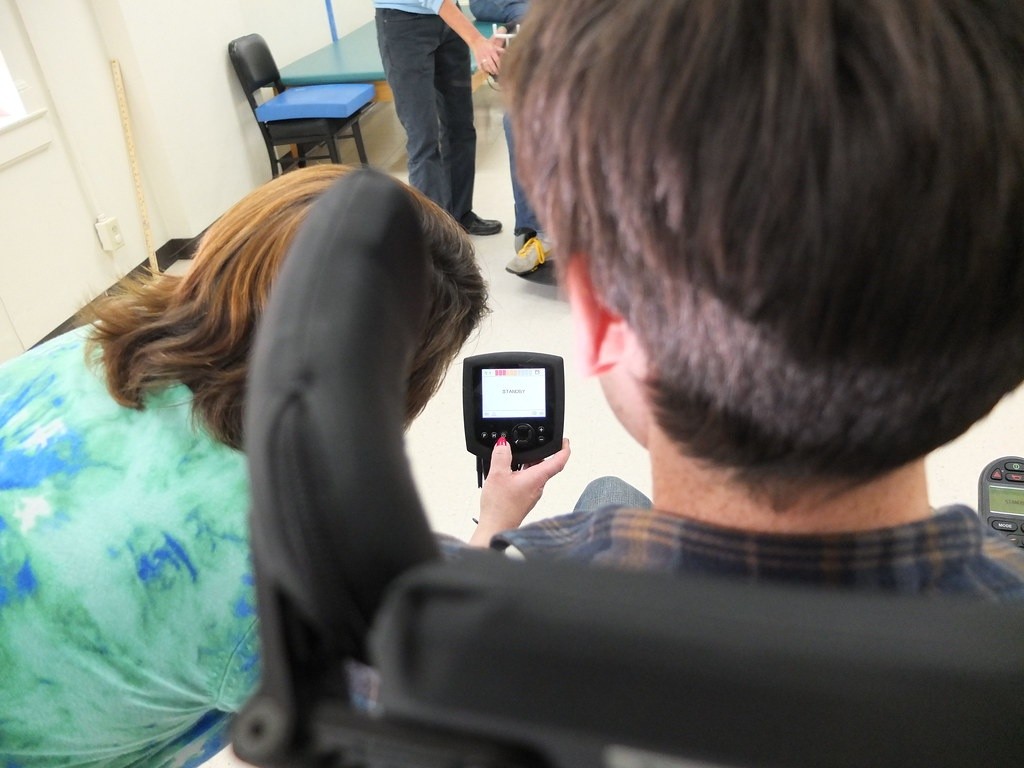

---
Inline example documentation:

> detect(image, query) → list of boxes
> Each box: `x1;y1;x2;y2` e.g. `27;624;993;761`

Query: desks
263;5;507;172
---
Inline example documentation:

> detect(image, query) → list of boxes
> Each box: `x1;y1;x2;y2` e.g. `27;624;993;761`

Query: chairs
228;33;369;181
230;170;1022;767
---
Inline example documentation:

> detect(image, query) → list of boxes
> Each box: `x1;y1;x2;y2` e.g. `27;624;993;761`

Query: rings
483;59;486;63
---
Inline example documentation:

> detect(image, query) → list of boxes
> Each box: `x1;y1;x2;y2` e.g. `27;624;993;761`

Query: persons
342;2;1024;768
0;166;570;767
376;0;506;235
469;0;556;275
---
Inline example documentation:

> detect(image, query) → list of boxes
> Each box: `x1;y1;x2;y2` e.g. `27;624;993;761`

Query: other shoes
462;212;502;235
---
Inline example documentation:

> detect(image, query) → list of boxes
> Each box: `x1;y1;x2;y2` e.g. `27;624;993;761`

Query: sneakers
505;236;554;278
